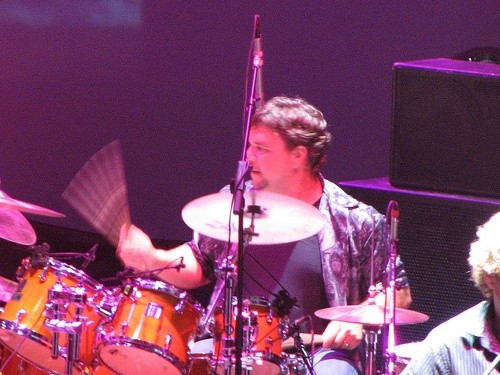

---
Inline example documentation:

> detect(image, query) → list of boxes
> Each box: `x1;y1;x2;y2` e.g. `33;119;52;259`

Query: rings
344;341;348;346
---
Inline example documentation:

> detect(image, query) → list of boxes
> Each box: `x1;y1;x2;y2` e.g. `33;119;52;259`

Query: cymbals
180;190;326;246
313;304;430;326
0;190;67;219
0;276;19;304
0;206;37;245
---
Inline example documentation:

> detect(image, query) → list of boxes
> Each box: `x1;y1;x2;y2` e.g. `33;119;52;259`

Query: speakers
335;176;500;347
389;56;500;199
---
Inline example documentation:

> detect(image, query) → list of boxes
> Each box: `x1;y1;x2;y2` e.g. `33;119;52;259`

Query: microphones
253;27;263;110
82;245;96;267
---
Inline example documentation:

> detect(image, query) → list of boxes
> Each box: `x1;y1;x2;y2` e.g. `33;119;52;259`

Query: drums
0;257;115;375
209;297;291;375
284;353;307;375
98;278;207;375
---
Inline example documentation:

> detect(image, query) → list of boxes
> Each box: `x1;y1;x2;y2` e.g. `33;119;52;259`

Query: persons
399;212;500;375
115;96;413;375
